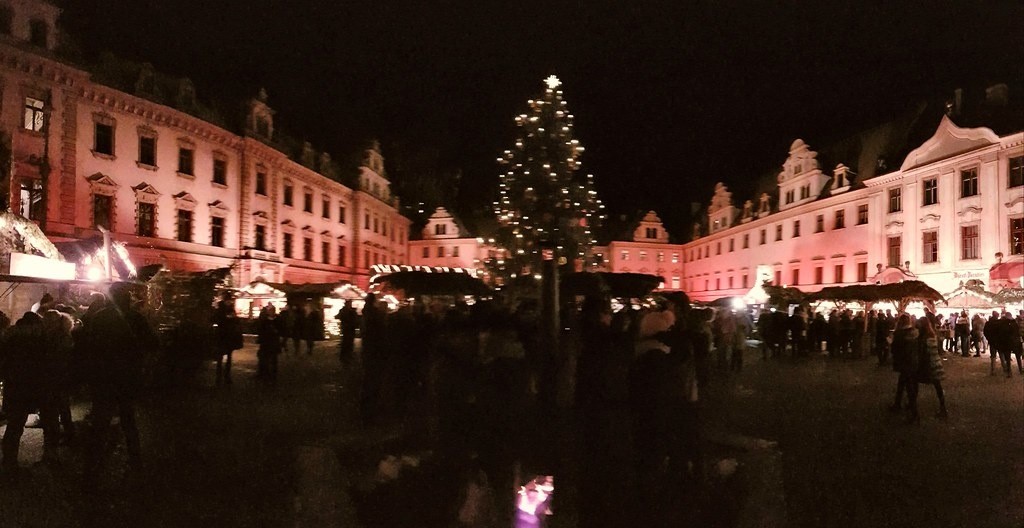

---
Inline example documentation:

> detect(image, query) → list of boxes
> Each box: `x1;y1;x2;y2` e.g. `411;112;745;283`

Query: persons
891;315;922;422
938;312;1024;379
759;309;893;362
214;298;236;377
915;318;946;417
0;292;153;460
335;296;744;528
253;303;325;374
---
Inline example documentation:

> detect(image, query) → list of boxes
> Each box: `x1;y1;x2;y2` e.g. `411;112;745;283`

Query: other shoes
906;414;921;425
905;401;920;409
888;404;901;410
935;409;948;417
